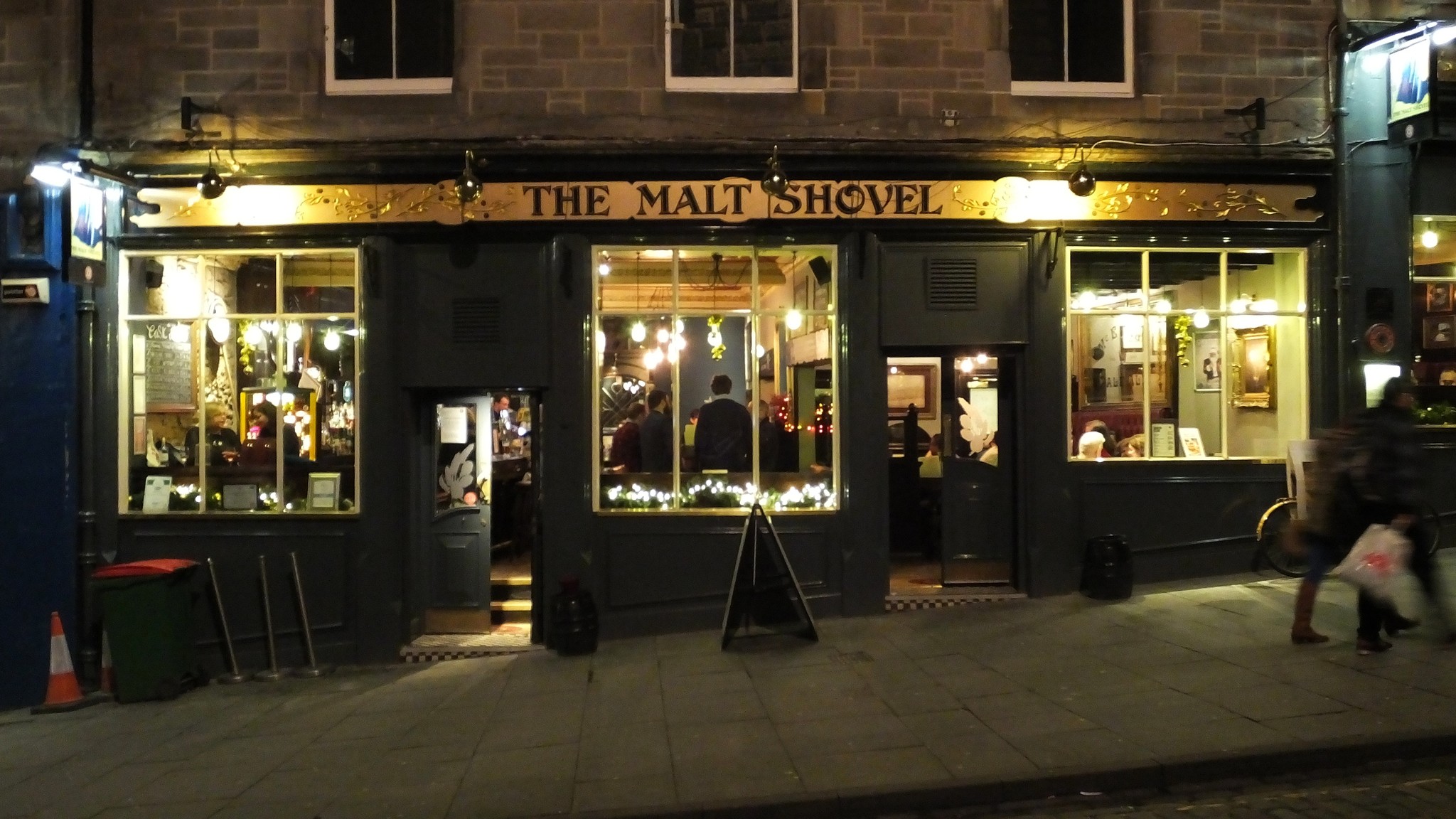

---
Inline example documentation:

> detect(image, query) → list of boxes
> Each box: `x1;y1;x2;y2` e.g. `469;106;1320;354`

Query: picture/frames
1192;331;1220;392
1229;325;1277;410
144;320;198;413
1422;315;1456;349
1426;283;1453;312
1120;326;1143;352
306;472;340;511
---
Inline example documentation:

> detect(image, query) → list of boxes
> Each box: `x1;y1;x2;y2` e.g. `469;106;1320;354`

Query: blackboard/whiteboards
142;316;200;414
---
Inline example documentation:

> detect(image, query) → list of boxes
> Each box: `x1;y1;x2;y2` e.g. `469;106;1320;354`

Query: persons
638;389;674;472
694;373;753;473
221;399;300;459
1084;368;1107;404
609;401;648;473
919;433;960;478
1287;379;1374;645
1072;417;1147;462
979;430;998;467
437;408;476;466
1202;347;1222;389
183;401;242;467
1339;377;1456;656
491;391;510;455
679;408;699;447
747;398;780;472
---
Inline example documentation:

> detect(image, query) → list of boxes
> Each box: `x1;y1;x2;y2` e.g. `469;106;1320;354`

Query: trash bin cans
84;558;206;704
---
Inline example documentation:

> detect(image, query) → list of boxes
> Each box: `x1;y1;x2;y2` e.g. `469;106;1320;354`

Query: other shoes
1356;635;1392;655
1291;628;1329;644
1384;615;1421;636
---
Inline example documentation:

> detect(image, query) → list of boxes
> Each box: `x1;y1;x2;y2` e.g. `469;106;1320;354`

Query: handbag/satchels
1326;523;1413;598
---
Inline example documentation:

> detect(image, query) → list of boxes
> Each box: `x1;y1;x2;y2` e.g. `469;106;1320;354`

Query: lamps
33;143;136;186
197;148;241;198
1055;143;1097;197
454;149;483;201
761;145;790;196
